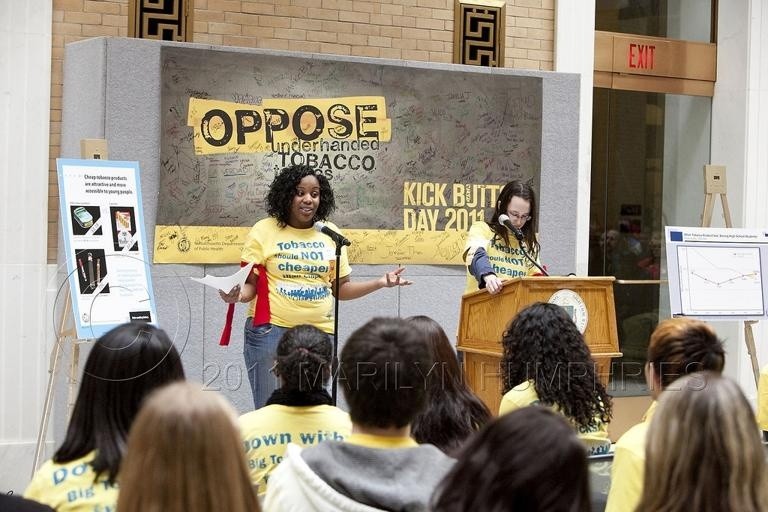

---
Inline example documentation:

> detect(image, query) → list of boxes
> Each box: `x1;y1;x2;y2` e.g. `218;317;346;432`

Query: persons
219;164;416;406
0;299;768;512
589;217;662;387
456;180;542;370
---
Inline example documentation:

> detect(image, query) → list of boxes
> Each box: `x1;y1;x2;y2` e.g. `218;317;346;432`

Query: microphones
499;214;522;239
313;220;351;246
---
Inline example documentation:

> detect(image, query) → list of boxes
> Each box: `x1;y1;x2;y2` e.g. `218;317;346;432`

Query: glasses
507;209;532;221
